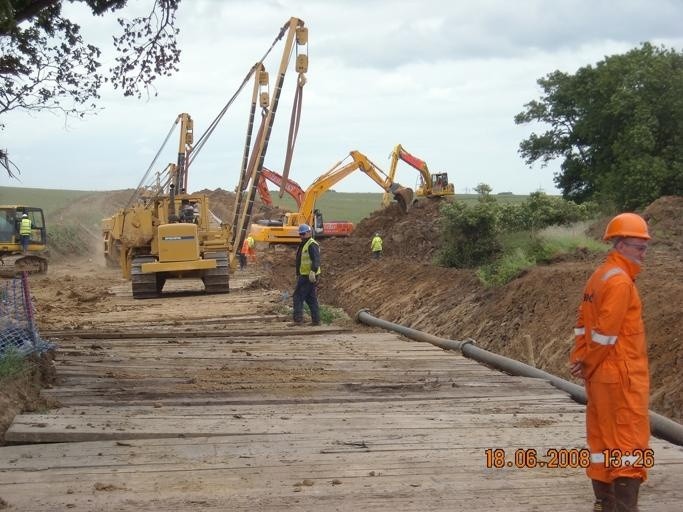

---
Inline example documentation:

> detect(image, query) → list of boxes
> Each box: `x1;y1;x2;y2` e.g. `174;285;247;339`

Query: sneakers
286;320;322;328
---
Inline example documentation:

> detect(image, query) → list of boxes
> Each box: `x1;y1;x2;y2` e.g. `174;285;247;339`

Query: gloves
309;270;316;284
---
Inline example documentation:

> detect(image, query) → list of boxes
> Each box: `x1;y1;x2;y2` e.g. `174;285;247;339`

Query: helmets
603;212;653;242
297;224;312;233
21;214;28;217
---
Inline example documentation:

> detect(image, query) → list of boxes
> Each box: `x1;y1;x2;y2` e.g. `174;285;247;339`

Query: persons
240;239;249;269
246;233;256;261
17;214;35;255
285;223;321;327
178;199;196;224
567;212;655;512
369;232;383;258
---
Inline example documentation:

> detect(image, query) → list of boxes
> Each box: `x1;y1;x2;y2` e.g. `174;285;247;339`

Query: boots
591;479;614;512
614;477;642;512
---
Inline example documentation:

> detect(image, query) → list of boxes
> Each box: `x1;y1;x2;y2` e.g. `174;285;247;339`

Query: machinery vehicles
249;146;454;244
0;205;48;278
